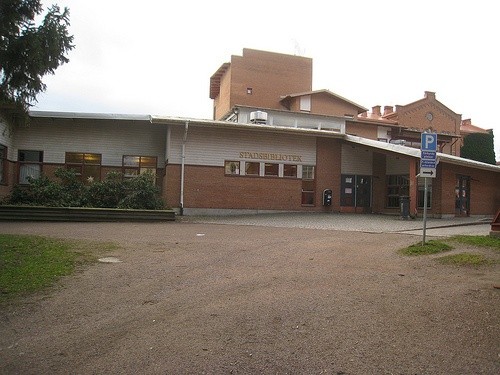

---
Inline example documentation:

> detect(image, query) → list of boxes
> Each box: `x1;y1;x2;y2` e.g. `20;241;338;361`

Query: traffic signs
420;151;435;178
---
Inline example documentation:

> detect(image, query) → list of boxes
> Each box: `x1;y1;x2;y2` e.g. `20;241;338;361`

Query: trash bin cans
399;196;411;217
322;188;333;206
